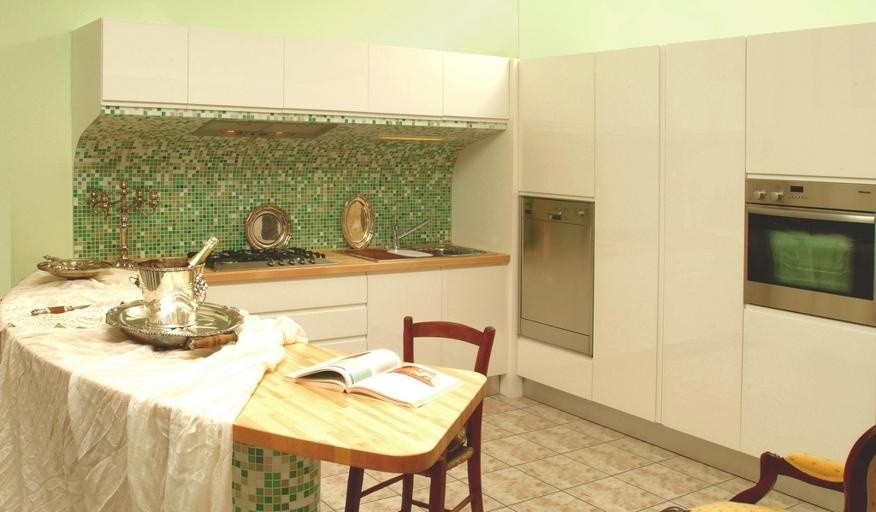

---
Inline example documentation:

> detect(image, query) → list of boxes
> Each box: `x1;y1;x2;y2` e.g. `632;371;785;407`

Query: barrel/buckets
135;258;208;331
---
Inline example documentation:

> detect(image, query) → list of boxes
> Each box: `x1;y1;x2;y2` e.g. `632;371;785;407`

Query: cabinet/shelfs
202;266;507;401
69;17;512;122
516;25;876;509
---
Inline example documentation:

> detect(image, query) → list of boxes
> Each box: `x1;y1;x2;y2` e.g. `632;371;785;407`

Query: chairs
342;317;497;512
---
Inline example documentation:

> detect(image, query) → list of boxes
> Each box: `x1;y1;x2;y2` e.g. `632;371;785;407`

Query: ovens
514;193;598;357
740;173;876;328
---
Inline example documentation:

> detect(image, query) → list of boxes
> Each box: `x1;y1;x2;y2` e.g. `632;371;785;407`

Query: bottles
181;235;219;267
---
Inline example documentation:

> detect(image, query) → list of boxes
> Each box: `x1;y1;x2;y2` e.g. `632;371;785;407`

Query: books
283;346;462;409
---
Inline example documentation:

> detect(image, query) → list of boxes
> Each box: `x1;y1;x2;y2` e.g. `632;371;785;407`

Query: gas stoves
188;246;337;273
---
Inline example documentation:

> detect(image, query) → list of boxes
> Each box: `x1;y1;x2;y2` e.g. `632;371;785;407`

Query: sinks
388;250;433;258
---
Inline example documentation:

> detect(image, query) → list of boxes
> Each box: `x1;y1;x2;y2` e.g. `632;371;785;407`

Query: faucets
391;219;432;249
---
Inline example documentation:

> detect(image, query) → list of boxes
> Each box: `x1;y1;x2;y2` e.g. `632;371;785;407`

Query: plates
105;297;246;351
37;259;115;280
